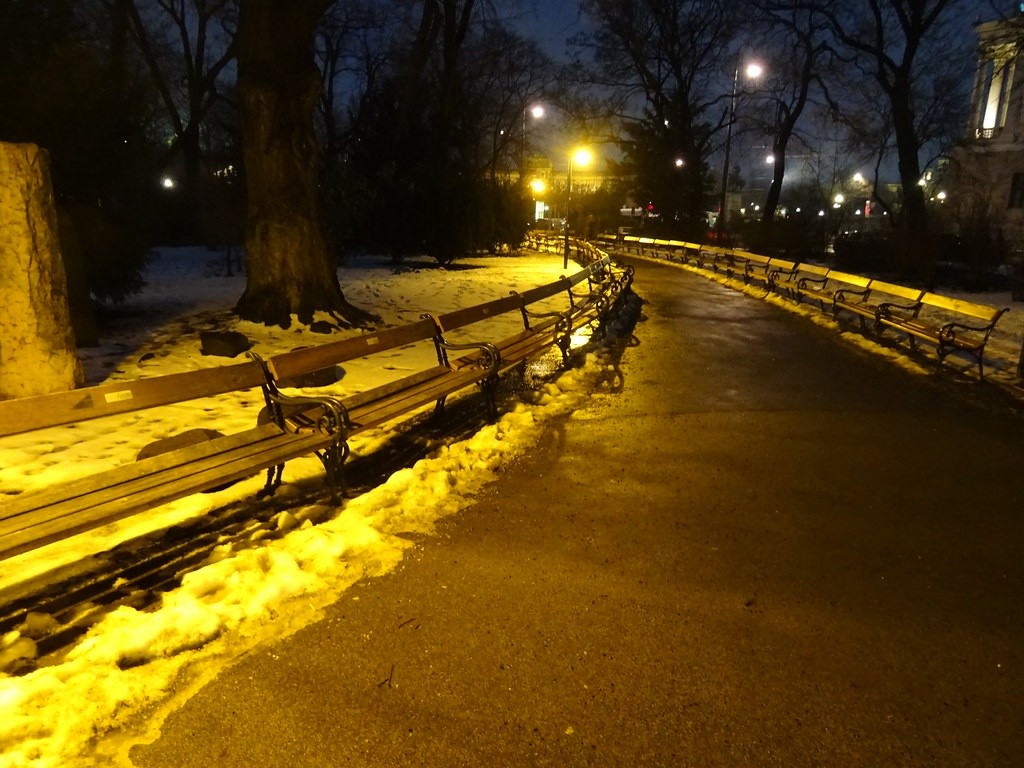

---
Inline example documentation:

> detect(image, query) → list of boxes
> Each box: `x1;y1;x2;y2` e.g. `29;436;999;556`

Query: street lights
564;145;594;269
716;41;766;248
519;103;545;187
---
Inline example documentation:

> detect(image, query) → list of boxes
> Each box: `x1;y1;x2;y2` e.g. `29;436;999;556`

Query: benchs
1;228;1010;561
